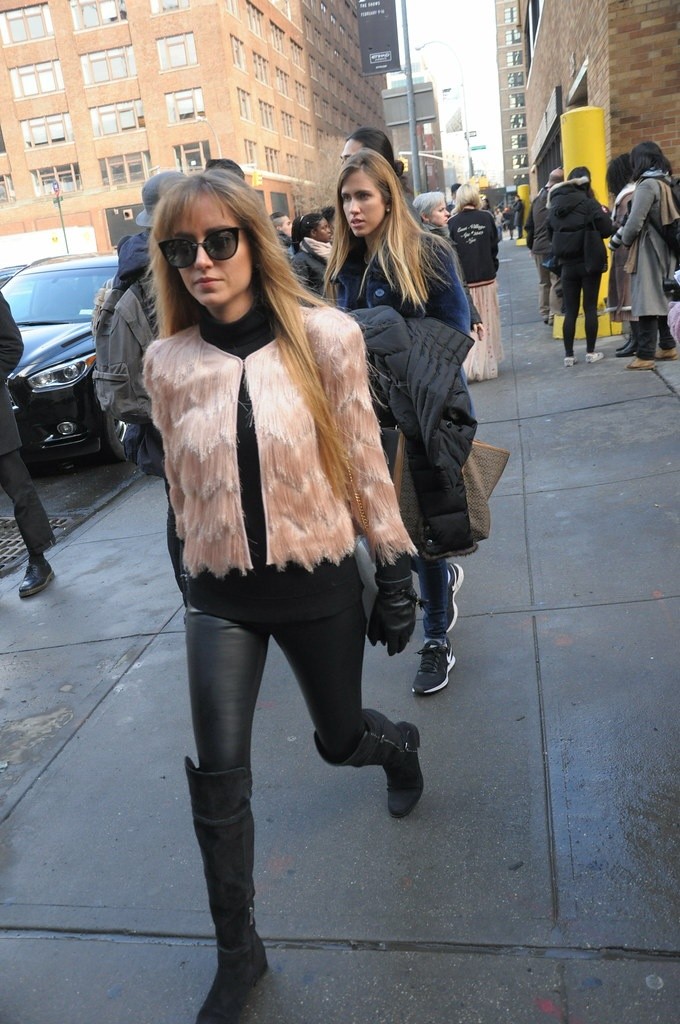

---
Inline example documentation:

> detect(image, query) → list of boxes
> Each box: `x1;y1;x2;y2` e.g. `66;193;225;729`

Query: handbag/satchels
583;230;608;274
382;425;511;545
541;255;561;278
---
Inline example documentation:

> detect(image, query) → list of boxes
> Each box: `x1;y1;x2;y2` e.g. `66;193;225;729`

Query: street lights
415;40;474;178
195;117;222;159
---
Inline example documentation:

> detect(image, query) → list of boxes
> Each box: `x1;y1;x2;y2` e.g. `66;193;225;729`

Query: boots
615;331;638;357
313;709;424;818
185;756;267;1024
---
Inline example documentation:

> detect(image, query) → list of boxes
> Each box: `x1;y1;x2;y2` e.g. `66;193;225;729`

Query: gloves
367;572;429;656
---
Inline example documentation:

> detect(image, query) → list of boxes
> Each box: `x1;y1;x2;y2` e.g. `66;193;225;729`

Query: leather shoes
655;348;679;360
19;559;55;597
627;358;655;370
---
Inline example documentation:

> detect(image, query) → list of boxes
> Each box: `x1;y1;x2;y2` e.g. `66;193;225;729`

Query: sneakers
446;563;464;633
412;636;456;693
564;357;577;366
586;352;604;363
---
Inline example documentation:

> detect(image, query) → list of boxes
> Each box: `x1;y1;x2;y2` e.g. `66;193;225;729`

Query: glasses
158;226;246;269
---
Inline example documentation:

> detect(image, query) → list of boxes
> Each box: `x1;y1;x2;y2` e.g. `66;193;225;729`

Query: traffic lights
253;170;262;187
400;157;408;174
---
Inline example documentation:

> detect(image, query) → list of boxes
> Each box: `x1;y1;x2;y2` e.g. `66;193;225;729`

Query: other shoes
544;319;553;326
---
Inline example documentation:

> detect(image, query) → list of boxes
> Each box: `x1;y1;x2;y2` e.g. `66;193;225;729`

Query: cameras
609;214;630;251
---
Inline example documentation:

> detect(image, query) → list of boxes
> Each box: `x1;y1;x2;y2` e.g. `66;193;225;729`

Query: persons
1;292;54;600
92;128;680;1024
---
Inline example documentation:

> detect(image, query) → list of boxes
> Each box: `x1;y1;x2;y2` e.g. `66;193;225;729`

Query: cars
1;257;128;474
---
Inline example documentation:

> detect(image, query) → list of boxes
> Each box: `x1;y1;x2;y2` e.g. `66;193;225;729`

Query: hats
544;169;564;187
136;171;187;227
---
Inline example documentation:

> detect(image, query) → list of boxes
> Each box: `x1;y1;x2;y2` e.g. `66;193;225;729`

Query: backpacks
92;271;157;424
637;176;680;260
